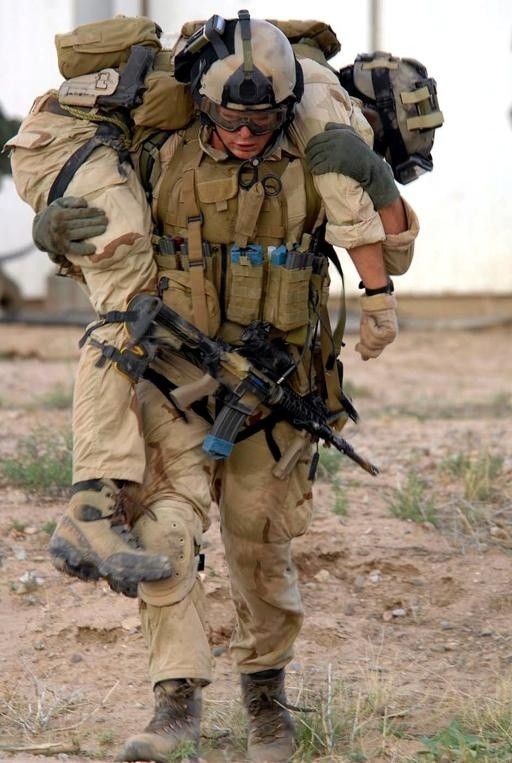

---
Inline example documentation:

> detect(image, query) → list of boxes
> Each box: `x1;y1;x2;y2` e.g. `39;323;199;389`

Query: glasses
369;66;435;183
193;90;293;135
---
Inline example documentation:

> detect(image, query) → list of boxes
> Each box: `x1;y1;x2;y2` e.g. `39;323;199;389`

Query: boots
125;676;213;760
46;478;174;598
240;674;316;763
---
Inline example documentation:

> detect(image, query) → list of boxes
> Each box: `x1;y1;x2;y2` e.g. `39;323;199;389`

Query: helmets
341;52;445;185
174;9;304;113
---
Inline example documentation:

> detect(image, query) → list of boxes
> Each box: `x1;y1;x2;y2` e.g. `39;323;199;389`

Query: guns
96;44;158;107
127;296;380;481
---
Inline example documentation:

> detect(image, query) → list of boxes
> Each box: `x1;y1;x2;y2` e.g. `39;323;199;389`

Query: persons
3;7;442;594
22;16;419;763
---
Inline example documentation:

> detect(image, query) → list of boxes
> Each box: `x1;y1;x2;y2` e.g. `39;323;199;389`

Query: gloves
304;120;399;212
32;195;112;267
353;295;400;361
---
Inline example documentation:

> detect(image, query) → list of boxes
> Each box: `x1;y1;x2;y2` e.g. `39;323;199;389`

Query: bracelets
359;278;395;293
